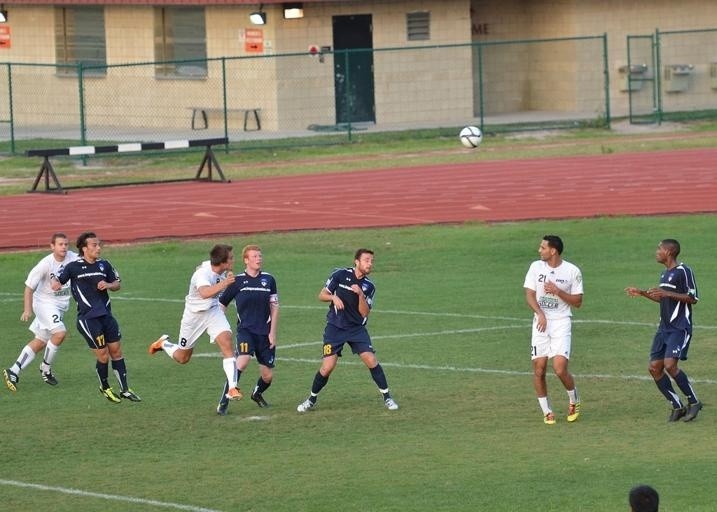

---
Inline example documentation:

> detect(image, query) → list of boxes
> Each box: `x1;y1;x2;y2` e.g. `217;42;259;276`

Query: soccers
459;126;482;148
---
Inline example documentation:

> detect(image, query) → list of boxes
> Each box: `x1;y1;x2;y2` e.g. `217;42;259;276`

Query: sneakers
567;398;581;422
225;386;242;400
684;398;702;422
251;392;267;408
40;363;57;385
149;334;170;354
543;409;555;424
99;385;121;403
120;388;141;401
297;399;317;412
669;407;686;421
217;397;229;414
3;369;19;391
384;398;398;410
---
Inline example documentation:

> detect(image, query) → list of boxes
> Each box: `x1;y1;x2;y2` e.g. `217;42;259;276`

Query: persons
624;238;703;422
215;245;280;416
148;243;244;402
628;484;659;511
522;235;584;424
297;248;398;413
50;232;141;403
2;232;81;391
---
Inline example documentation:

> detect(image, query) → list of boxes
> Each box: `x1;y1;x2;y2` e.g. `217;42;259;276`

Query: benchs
187;104;261;132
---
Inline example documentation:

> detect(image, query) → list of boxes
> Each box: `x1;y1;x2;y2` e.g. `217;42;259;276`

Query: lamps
280;2;304;19
248;4;266;25
0;3;8;22
